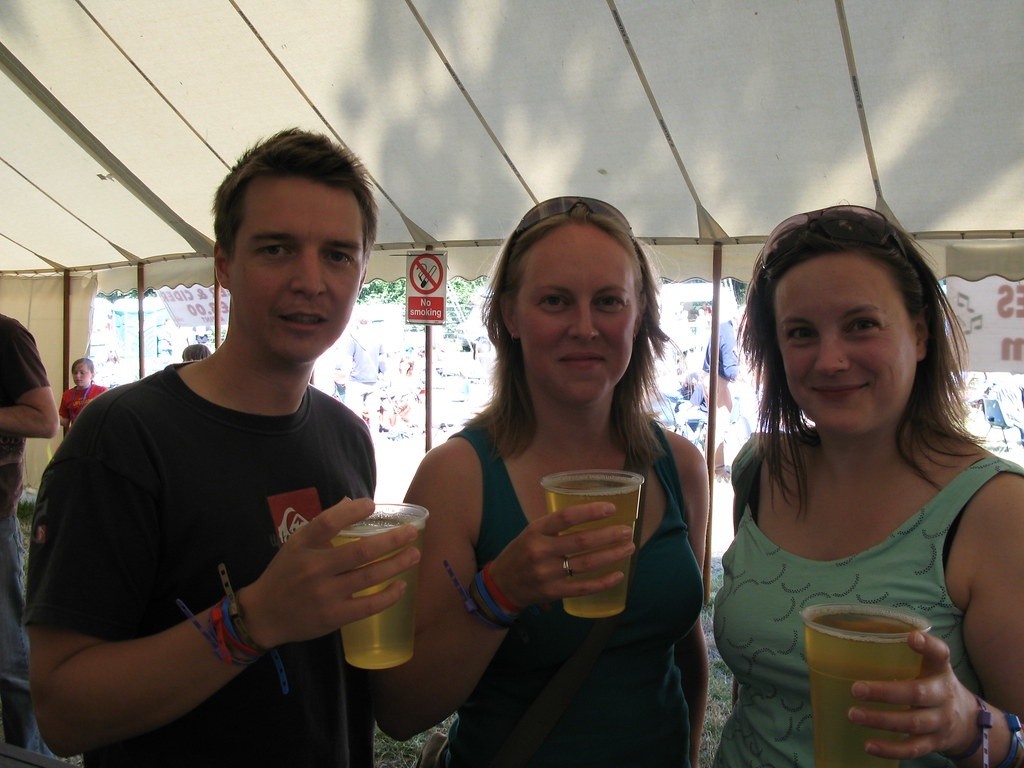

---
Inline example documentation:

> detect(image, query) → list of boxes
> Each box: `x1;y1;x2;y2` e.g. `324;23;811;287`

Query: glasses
760;204;908;270
514;196;636;244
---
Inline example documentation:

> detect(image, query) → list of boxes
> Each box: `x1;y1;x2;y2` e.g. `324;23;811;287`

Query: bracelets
444;559;525;630
937;697;1024;768
176;561;268;667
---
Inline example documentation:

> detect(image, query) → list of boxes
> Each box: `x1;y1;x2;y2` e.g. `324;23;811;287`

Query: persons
183;344;211;361
0;314;51;758
710;205;1024;768
20;126;422;768
60;358;109;438
374;196;712;768
687;303;747;483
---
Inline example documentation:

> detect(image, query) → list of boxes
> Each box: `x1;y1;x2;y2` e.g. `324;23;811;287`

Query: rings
562;556;574;576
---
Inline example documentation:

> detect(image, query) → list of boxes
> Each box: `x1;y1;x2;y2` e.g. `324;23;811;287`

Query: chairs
982;398;1020;454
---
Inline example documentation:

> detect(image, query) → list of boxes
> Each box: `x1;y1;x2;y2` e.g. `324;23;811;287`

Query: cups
542;469;645;618
330;504;429;669
798;603;932;767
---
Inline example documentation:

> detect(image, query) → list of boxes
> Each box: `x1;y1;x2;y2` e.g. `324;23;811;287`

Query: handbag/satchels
411;733;448;768
